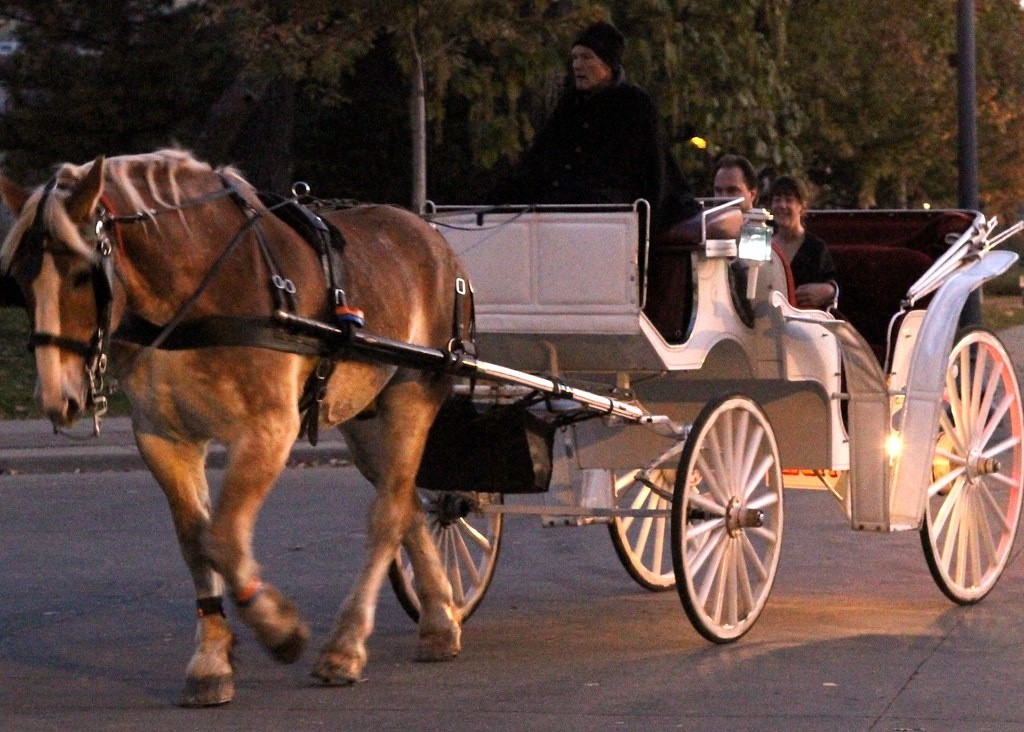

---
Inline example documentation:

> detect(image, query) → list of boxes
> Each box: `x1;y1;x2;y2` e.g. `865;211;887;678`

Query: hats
572;19;625;75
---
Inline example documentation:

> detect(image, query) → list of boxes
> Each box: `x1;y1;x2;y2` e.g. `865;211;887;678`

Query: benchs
641;194;986;438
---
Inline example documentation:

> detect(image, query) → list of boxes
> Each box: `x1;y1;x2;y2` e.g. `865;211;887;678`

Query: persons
766;175;828;311
515;20;701;290
712;154;840;311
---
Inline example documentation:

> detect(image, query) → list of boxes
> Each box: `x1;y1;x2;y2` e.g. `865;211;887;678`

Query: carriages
1;146;1023;706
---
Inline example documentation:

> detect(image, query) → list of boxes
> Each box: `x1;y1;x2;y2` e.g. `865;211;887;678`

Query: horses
0;146;476;705
